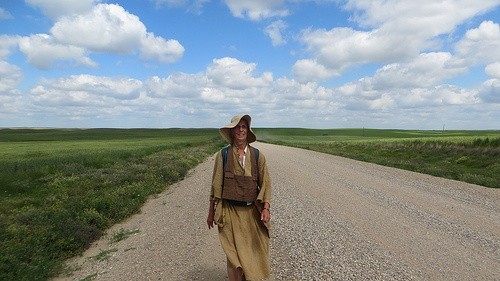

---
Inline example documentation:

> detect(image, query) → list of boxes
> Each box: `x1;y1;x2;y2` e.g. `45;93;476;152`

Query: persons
206;114;271;281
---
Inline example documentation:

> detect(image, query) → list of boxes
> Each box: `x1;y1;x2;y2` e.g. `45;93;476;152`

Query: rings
267;219;268;222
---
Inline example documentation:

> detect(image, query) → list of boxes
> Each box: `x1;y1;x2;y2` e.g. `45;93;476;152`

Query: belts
223;199;255;207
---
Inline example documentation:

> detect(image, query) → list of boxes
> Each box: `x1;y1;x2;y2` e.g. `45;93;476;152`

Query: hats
219;114;256;145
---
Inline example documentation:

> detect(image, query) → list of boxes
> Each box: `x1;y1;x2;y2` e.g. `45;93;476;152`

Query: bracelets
264;208;270;212
